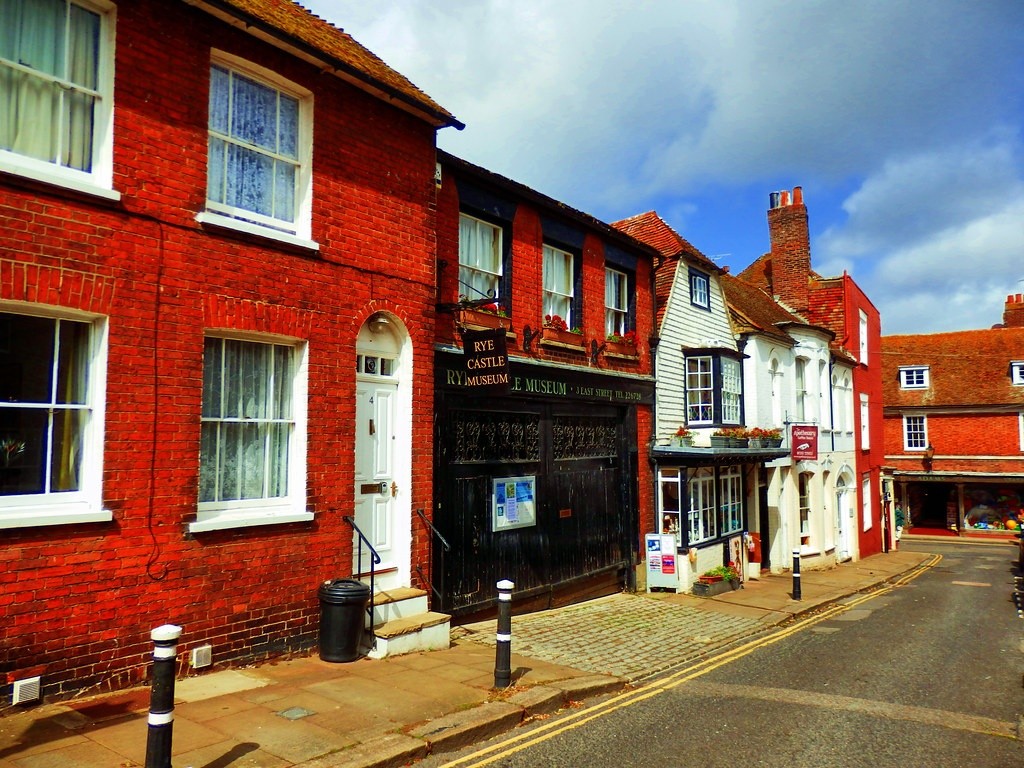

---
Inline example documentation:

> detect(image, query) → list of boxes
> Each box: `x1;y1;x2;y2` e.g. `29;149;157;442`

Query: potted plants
699;569;722;584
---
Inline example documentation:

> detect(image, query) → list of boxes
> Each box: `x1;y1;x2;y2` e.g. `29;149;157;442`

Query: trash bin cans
317;579;372;663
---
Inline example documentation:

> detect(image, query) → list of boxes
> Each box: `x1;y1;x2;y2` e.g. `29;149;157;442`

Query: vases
677;436;692;447
771;438;783;448
747;439;760;449
542;327;583;346
605;343;637;356
693;578;741;596
459;310;511;332
761;438;768;448
710;436;730;448
729;437;748;447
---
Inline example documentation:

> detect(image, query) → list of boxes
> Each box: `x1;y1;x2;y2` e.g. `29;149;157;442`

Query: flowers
719;561;743;582
543;315;584;335
674;426;691;437
458;294;506;317
607;330;635;345
713;427;782;440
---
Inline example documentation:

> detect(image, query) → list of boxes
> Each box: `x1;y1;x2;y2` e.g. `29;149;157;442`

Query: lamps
926;443;934;467
